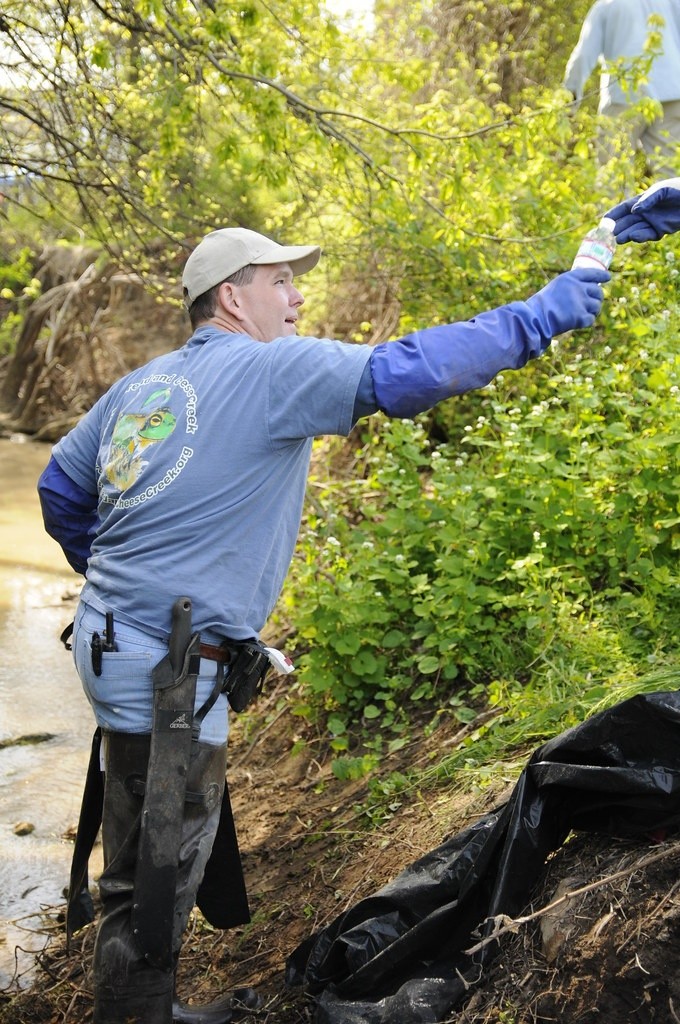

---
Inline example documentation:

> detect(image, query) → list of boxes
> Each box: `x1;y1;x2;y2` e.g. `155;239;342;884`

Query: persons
604;177;680;244
547;0;680;202
36;229;612;1024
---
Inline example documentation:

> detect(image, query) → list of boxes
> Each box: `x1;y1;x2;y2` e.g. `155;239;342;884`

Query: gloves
39;455;96;576
603;176;680;242
369;267;612;418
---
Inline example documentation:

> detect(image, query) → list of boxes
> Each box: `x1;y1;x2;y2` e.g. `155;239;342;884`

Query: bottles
570;217;618;286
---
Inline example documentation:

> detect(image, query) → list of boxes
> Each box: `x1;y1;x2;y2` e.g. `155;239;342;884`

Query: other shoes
172;988;261;1024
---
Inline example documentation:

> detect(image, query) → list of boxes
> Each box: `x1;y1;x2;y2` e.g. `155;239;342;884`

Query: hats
182;227;321;311
